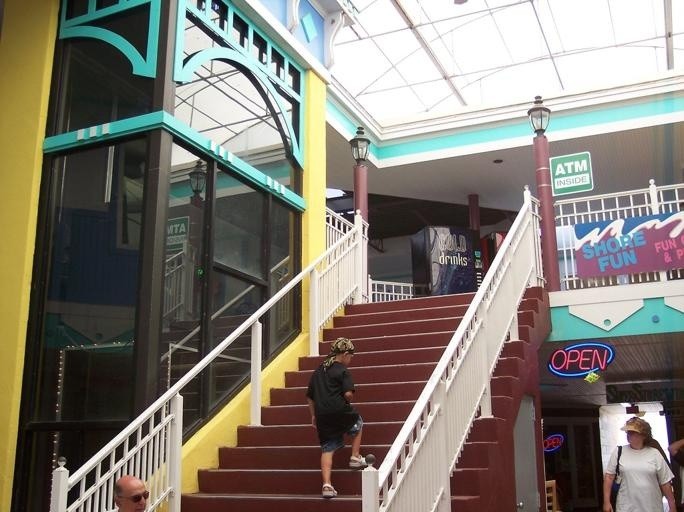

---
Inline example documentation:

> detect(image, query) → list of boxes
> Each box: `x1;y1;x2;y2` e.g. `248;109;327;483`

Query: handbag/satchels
609;446;623;512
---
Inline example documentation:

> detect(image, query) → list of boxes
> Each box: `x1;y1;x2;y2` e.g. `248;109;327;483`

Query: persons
601;416;677;512
112;474;150;512
303;335;374;498
667;437;684;512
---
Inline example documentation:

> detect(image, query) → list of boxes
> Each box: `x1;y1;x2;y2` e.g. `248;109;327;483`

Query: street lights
187;159;207;317
349;126;370;224
528;95;561;292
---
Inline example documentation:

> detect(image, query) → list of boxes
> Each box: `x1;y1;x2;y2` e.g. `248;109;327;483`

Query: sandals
348;454;368;469
321;484;339;499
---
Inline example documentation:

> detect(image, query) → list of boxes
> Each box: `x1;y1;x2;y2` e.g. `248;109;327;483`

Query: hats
619;421;648;434
